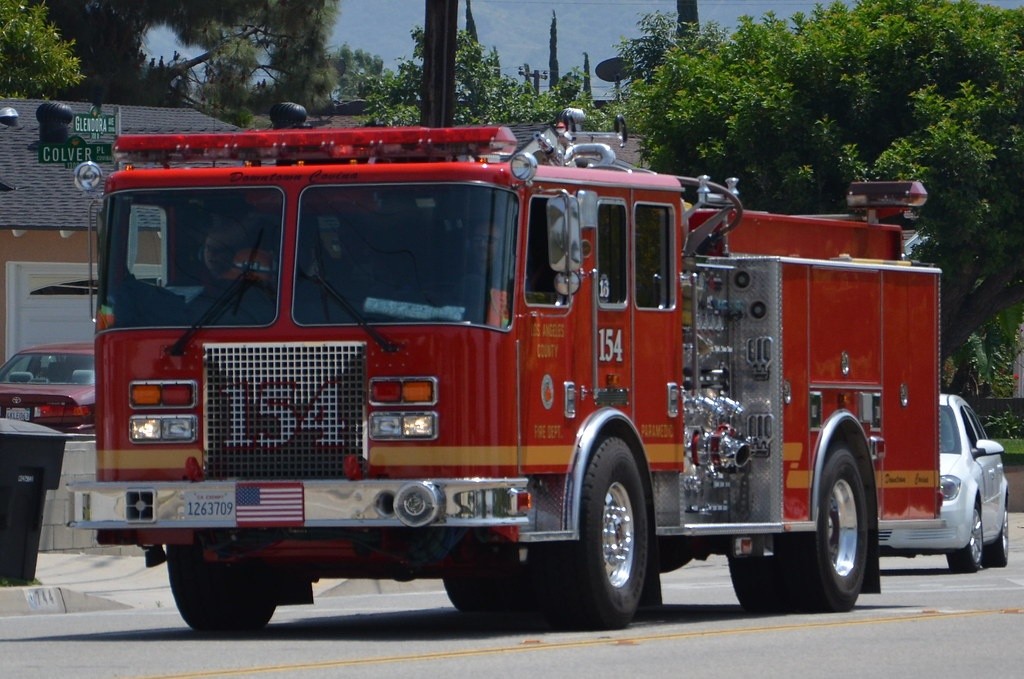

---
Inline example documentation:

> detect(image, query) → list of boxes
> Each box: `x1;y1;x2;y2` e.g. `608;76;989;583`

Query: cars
878;394;1010;574
0;340;96;435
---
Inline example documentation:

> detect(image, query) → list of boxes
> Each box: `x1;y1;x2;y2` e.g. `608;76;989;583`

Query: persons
190;225;238;300
449;217;513;310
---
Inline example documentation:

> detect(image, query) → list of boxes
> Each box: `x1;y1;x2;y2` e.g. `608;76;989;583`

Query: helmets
221;249;275;284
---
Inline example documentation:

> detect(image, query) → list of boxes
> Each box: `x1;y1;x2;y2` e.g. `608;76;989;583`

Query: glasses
470;234;498;243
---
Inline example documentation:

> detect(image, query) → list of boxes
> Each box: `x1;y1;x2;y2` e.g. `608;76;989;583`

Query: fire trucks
64;102;944;632
